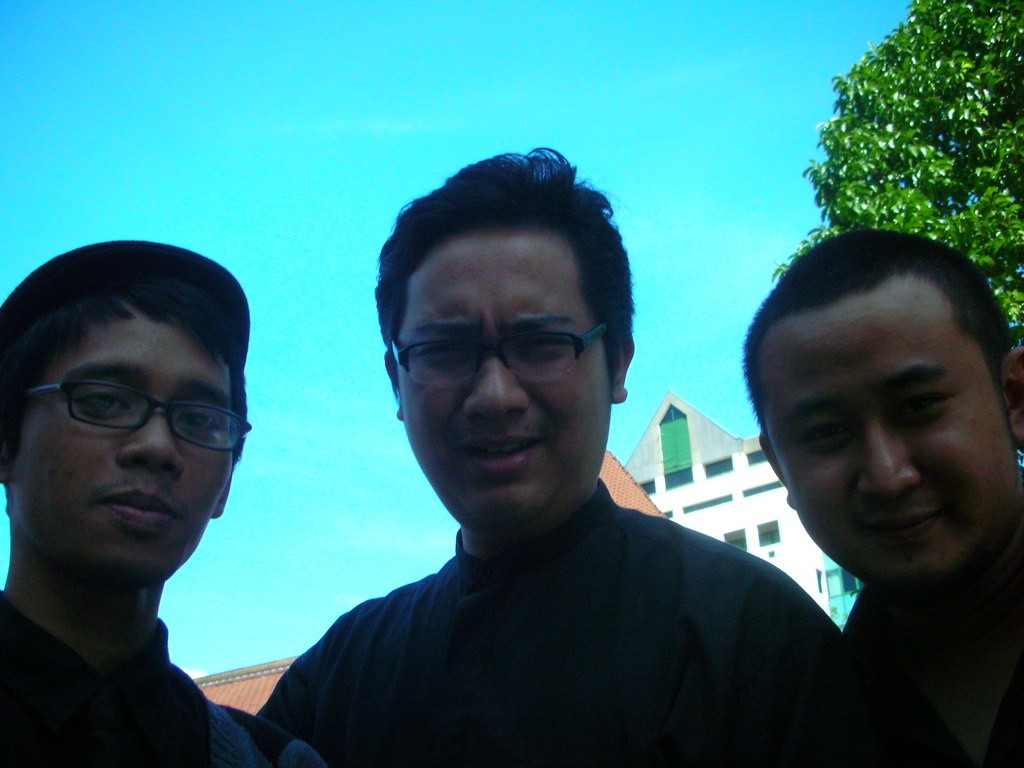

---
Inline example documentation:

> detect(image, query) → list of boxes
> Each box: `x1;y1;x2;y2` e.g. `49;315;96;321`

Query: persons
255;148;850;768
744;227;1023;767
0;240;328;767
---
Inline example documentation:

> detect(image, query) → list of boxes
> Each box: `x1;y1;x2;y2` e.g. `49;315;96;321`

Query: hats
0;241;251;373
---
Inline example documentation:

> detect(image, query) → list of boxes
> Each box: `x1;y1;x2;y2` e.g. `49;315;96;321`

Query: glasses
21;380;252;449
390;322;607;384
